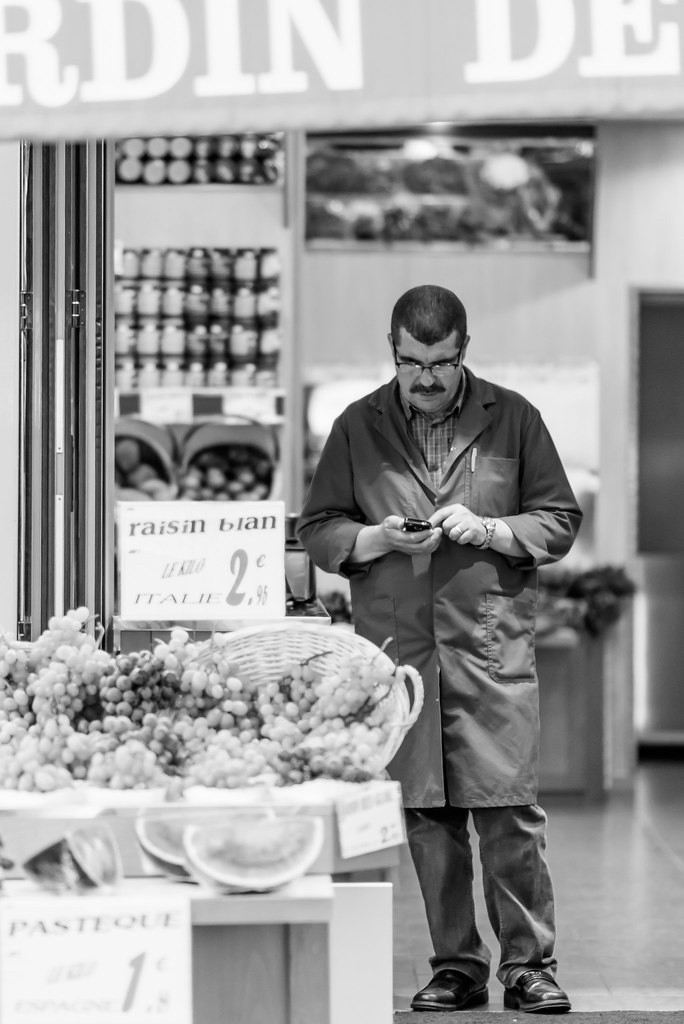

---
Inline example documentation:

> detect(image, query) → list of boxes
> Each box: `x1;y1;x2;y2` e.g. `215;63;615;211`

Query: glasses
392;336;464;377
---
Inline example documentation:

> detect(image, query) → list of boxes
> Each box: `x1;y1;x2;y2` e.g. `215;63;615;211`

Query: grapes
1;605;406;798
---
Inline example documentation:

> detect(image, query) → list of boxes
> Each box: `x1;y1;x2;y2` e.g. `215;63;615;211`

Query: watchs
474;516;496;551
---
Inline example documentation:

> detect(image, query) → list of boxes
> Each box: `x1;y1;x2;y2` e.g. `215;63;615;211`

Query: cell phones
404;517;432;531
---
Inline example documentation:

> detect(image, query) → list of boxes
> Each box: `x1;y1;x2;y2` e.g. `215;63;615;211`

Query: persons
295;285;584;1013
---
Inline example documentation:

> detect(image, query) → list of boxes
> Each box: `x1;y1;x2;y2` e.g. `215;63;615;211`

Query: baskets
181;622;426;780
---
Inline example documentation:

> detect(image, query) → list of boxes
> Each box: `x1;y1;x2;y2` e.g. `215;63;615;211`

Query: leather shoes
503;971;573;1014
409;970;489;1012
